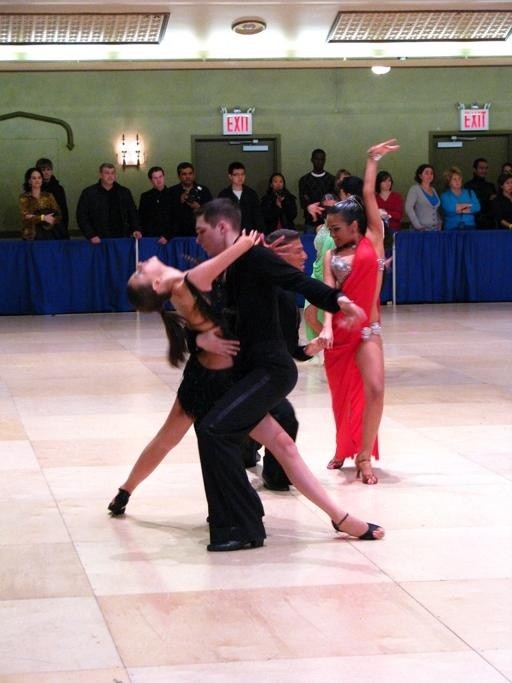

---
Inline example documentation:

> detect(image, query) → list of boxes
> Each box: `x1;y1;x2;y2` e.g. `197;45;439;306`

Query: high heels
327;454;354;469
107;488;130;518
355;454;378;485
332;513;385;540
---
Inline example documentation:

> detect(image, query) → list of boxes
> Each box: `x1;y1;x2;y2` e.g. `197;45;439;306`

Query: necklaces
345;243;356;250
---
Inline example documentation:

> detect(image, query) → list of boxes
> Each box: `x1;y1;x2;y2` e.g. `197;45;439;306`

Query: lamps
114;132;145;171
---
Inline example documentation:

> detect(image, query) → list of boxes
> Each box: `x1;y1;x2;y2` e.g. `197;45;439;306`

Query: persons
216;163;263;236
36;157;70;235
108;229;386;540
19;169;66;241
312;137;402;487
241;229;323;492
171;161;214;239
298;147;512;229
259;171;297;232
175;199;367;554
337;176;394;263
76;163;143;245
137;166;180;243
300;194;371;347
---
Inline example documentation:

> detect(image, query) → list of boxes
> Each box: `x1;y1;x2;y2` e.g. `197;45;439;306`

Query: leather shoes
207;536;264;551
263;482;289;491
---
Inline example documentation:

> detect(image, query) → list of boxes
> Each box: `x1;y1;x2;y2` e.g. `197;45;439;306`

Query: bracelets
40;214;45;221
